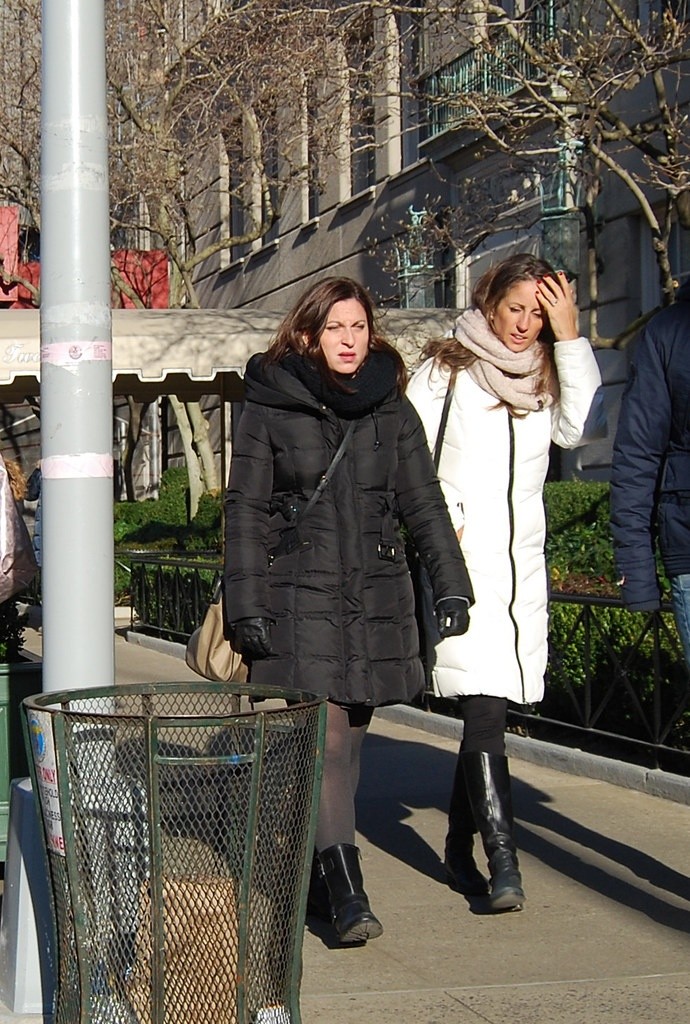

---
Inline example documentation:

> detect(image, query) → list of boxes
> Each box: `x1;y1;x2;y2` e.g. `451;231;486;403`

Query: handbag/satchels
185;574;251;686
399;531;444;648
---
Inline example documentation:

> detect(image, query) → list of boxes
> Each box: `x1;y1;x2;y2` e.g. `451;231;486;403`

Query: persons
221;276;478;945
609;280;689;665
403;252;603;911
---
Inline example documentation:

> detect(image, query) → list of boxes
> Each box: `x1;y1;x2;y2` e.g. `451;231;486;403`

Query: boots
318;842;385;949
459;750;525;912
307;847;333;925
442;741;489;897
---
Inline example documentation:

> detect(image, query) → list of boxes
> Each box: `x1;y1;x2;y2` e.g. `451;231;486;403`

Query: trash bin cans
19;680;333;1024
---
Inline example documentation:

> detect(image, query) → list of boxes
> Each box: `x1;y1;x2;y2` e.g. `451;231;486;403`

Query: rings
551;299;559;306
